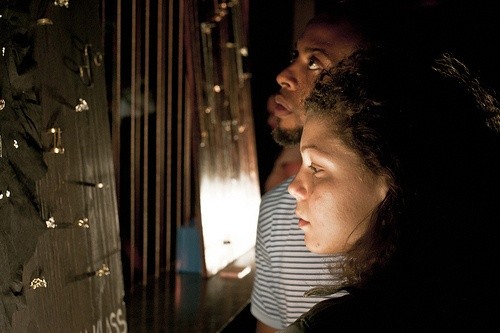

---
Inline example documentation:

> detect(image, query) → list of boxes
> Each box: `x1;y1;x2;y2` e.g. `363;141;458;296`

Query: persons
250;1;389;333
279;44;500;333
263;91;305;193
